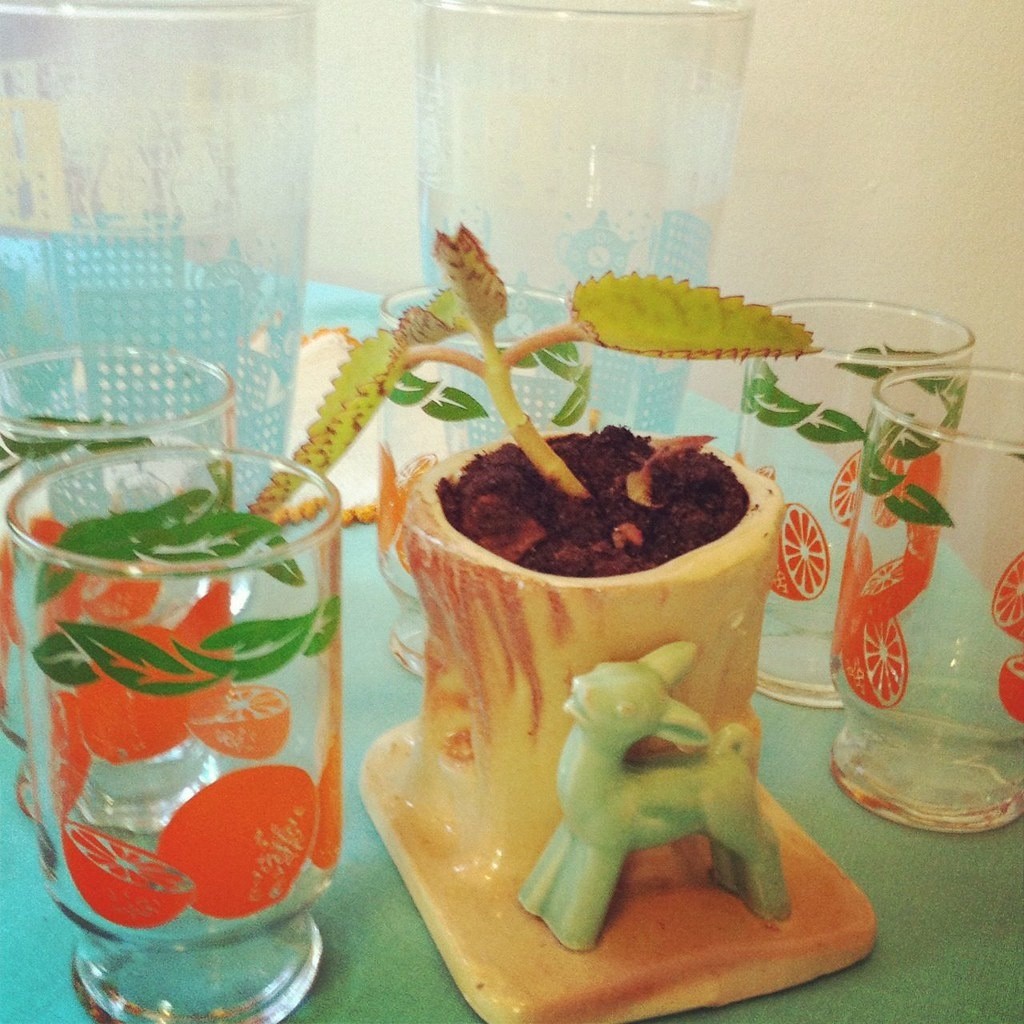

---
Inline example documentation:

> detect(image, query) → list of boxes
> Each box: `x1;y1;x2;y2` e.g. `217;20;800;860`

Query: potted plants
256;227;878;1023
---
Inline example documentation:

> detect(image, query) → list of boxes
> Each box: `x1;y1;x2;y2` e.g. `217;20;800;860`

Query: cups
416;0;754;461
831;367;1024;834
737;303;975;708
0;346;237;838
0;446;342;1024
1;0;314;470
377;289;593;680
